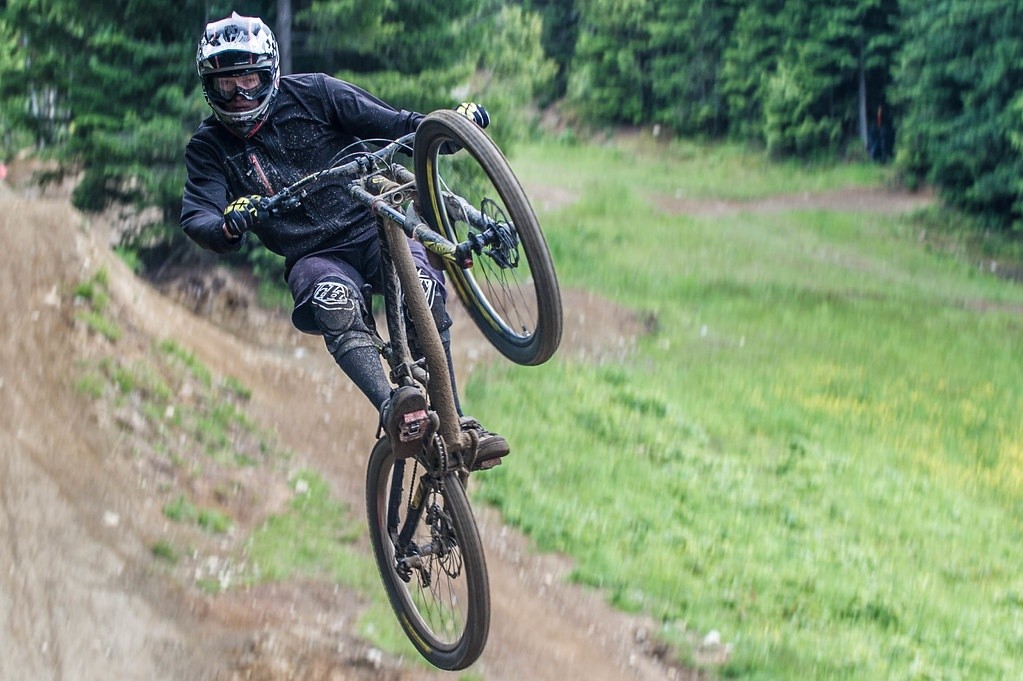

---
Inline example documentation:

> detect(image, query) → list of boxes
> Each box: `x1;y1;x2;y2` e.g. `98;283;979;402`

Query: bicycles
255;104;567;674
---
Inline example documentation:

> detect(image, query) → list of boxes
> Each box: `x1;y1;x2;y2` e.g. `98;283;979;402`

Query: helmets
196;10;280;140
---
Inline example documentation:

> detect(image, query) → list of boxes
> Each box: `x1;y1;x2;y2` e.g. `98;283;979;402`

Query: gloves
455;101;491;129
223;194;270;239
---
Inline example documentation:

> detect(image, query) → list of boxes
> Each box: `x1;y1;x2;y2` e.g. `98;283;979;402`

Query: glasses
204;68;274;103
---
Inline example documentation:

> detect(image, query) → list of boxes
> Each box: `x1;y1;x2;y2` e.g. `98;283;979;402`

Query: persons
180;11;510;471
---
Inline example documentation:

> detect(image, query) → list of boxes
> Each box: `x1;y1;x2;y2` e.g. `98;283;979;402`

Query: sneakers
374;386;427;460
458;415;510;465
